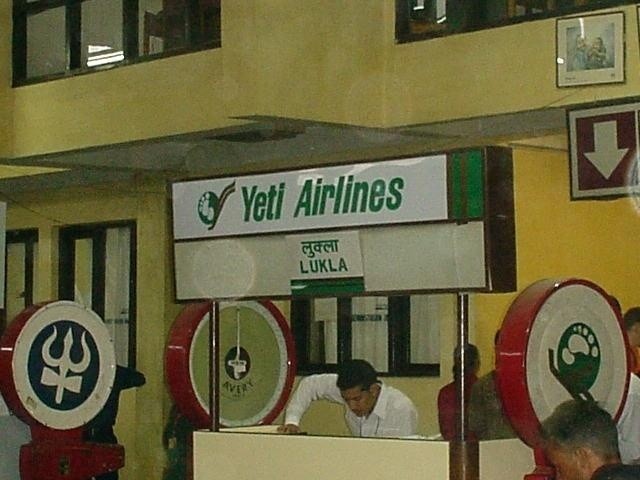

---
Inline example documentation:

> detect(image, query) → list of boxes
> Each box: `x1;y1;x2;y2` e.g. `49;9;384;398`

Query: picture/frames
636;6;640;45
556;11;626;89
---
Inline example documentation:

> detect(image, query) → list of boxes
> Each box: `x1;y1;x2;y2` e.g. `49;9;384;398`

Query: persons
276;359;418;438
587;38;607;70
437;344;483;441
468;327;518;440
539;398;640;480
566;34;592;73
612;305;640;467
